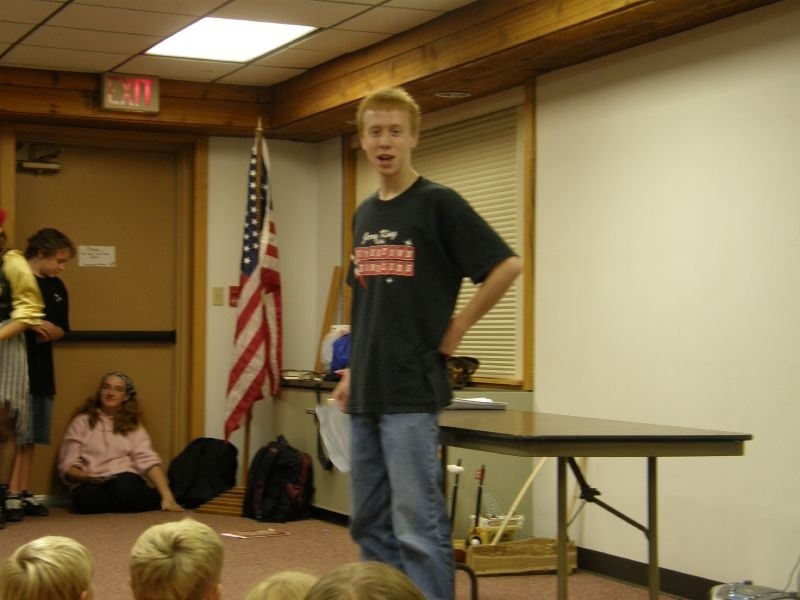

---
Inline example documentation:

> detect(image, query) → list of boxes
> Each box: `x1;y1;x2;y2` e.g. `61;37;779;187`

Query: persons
8;228;77;531
1;233;45;527
57;370;185;515
1;518;426;599
330;84;522;600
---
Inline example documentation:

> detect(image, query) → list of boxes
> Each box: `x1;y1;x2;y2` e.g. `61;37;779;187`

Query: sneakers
5;491;23;521
20;489;52;517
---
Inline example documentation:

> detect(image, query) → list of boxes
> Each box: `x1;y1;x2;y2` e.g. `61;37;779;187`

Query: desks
433;410;754;600
272;379;507;471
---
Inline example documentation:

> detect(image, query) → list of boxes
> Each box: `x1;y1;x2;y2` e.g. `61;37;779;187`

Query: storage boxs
467;538;577;575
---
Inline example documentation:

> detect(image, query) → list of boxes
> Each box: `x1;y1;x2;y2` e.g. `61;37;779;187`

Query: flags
224;135;284;443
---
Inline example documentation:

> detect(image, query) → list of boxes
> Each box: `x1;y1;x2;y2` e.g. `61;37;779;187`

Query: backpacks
243;440;316;522
169;436;238;508
